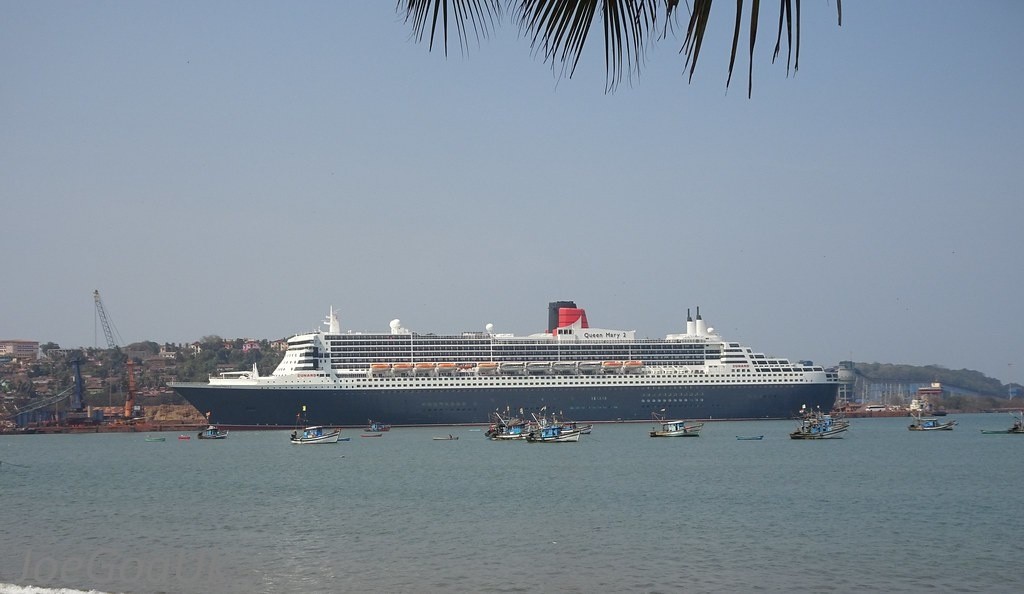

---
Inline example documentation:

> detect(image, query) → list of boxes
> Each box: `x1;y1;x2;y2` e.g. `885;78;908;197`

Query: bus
865;404;887;413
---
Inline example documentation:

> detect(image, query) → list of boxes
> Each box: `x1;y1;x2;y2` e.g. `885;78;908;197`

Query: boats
432;433;459;440
475;364;498;372
289;405;341;444
736;435;764;440
337;437;351;441
364;418;391;432
178;434;191;440
907;409;959;431
649;412;704;437
361;433;382;437
414;363;436;371
603;362;622;370
370;364;390;371
392;364;413;372
145;435;166;442
436;364;458;370
789;404;850;439
197;424;229;439
623;361;644;370
484;405;593;443
979;416;1024;435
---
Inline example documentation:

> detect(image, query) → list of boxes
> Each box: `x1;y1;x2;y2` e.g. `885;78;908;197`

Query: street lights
1007;364;1012;400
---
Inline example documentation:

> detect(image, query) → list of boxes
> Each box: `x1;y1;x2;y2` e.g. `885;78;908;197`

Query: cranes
93;289;126;350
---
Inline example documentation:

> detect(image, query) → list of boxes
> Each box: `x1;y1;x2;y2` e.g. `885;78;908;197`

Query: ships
165;301;840;429
